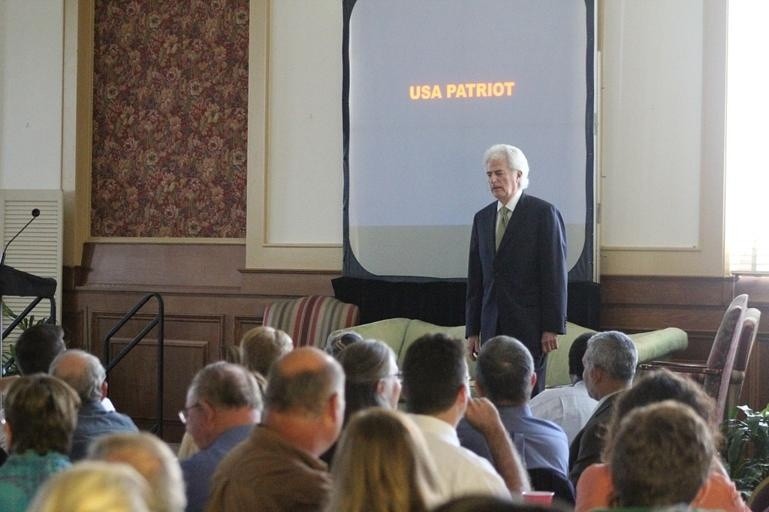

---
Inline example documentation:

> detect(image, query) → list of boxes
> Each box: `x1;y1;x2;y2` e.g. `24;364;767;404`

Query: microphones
1;209;40;263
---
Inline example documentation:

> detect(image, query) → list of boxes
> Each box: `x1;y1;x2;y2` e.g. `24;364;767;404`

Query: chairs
672;308;762;427
639;295;749;427
262;295;359;352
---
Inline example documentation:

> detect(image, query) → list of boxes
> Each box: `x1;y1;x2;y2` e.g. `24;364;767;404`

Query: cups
521;491;555;506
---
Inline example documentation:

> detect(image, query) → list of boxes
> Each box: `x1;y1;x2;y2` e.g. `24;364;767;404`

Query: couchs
327;319;688;410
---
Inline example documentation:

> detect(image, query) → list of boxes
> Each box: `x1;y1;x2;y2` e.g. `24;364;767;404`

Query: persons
0;374;78;512
322;339;401;468
87;432;188;511
28;464;149;511
14;323;66;374
457;336;571;476
528;332;600;440
328;406;446;512
567;331;638;475
324;331;364;354
608;403;714;511
0;374;24;453
573;366;753;511
206;346;345;512
49;349;140;460
400;333;532;511
176;325;294;467
465;142;569;398
175;359;263;511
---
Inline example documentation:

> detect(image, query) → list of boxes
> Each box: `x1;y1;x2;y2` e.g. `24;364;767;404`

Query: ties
496;207;509;251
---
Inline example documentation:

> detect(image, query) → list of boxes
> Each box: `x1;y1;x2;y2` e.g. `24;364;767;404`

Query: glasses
0;409;7;424
392;371;405;379
178;402;199;424
